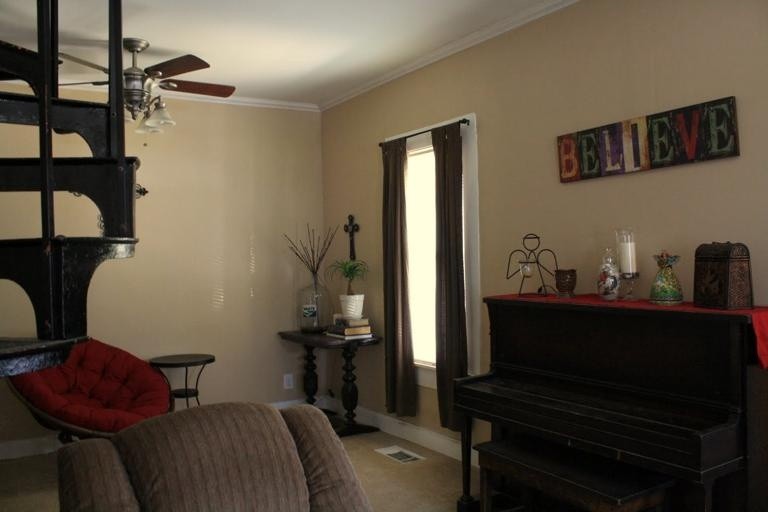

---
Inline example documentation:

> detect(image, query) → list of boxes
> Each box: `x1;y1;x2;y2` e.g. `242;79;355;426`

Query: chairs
58;401;373;512
5;339;174;443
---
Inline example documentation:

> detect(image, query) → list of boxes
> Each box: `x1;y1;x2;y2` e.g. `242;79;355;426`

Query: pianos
450;293;768;511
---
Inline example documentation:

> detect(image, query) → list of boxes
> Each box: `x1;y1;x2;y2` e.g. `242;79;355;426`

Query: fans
59;38;235;98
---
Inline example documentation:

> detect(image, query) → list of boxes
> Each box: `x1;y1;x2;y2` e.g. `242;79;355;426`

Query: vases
300;276;332;333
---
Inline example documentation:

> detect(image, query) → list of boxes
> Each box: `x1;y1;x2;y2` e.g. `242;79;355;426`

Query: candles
619;242;637;273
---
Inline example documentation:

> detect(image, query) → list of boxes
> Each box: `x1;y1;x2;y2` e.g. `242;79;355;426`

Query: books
328;325;372;336
336;317;369;327
325;331;373;340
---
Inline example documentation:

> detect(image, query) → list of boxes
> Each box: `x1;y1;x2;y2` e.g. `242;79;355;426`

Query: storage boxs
694;242;752;310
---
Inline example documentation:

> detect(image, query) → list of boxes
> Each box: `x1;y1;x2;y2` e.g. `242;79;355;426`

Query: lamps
123;95;176;134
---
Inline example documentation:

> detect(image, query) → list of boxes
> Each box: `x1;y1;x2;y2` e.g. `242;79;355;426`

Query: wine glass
614;229;640;301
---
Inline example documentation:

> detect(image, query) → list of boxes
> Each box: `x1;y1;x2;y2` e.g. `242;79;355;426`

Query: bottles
648;255;684;306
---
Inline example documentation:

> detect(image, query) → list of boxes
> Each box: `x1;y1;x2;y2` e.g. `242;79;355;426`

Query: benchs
473;437;677;511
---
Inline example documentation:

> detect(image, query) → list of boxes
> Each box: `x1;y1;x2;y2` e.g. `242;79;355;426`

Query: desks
280;331;383;437
149;354;215;408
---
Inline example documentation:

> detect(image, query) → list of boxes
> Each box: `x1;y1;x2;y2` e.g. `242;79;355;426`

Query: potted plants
325;258;369;320
